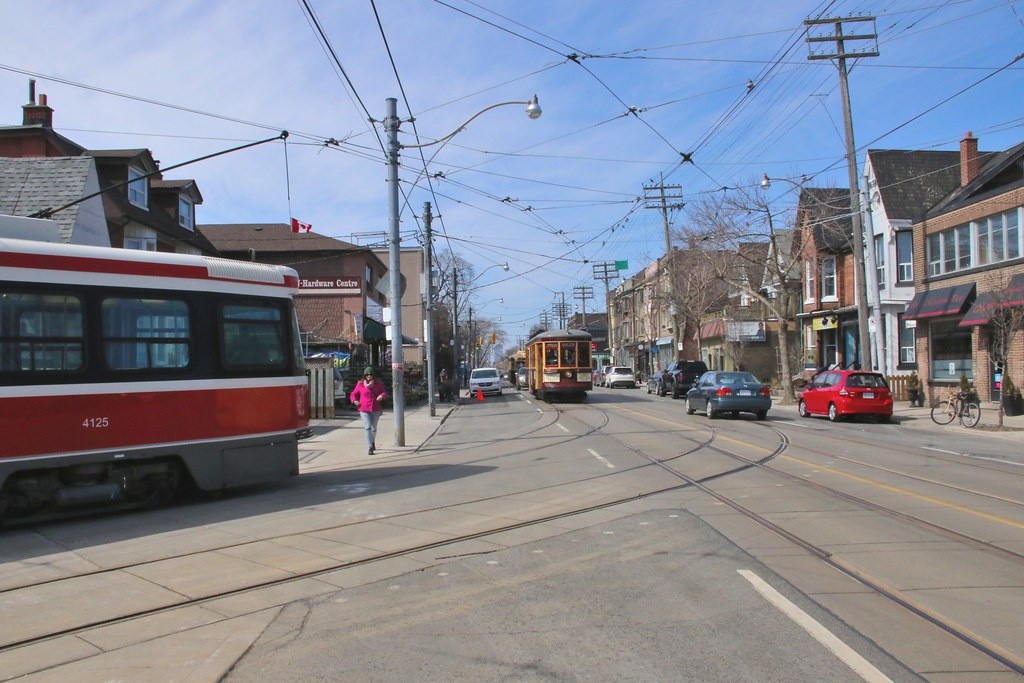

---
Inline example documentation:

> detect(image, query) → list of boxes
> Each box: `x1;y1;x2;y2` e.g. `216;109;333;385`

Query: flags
291;218;312;233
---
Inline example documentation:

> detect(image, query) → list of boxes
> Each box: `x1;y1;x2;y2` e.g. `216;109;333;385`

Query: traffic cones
476;387;486;403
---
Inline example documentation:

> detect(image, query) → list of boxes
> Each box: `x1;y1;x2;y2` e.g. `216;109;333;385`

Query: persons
757;322;765;335
350;366;389;454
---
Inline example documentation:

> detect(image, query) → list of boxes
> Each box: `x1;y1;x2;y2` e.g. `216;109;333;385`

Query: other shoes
373;446;375;450
368;449;373;454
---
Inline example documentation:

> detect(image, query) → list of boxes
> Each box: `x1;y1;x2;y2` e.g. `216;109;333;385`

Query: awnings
958;292;1002;326
993;273;1024;309
902;281;976;319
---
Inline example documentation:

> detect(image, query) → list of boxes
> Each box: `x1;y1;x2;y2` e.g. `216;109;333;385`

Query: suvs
659;359;709;399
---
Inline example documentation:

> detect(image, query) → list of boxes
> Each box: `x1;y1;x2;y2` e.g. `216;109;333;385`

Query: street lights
759;175;872;368
379;92;542;445
467;298;505;370
452;261;509;381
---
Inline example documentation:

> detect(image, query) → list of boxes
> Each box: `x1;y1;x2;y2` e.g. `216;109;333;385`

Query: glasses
365;374;372;376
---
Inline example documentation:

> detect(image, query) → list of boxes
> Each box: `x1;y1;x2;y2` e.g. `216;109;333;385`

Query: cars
594;365;637;388
515;367;529;390
798;370;894;423
685;370;772;421
646;371;663;395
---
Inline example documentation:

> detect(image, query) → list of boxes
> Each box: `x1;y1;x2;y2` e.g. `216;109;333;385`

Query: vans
469;367;503;398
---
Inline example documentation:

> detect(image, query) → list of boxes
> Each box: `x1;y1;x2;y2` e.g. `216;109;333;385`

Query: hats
364;367;374;376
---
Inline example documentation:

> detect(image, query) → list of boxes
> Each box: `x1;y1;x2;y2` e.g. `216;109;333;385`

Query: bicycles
929;384;981;428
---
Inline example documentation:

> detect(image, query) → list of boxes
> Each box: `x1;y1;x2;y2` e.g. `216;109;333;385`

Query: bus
496;353;526;381
525;326;593;400
1;131;316;523
